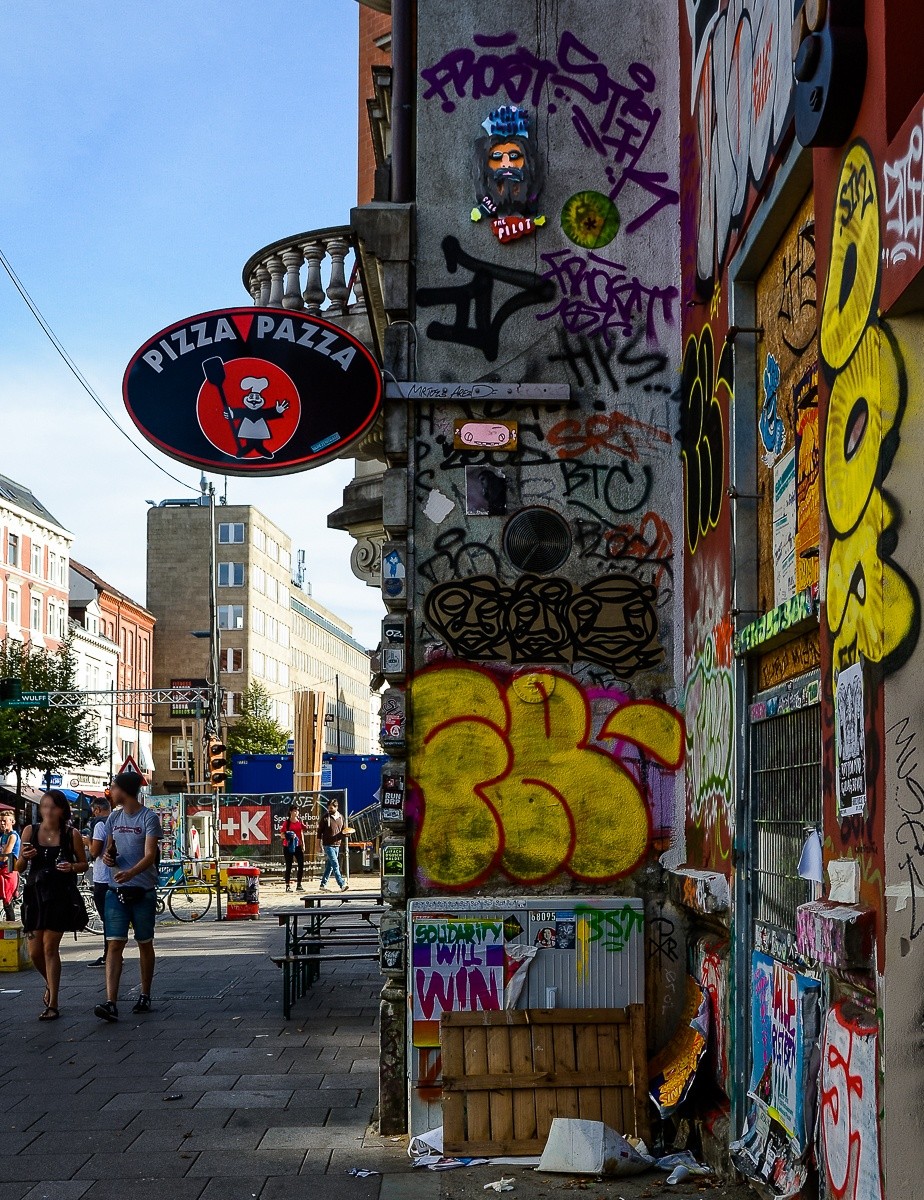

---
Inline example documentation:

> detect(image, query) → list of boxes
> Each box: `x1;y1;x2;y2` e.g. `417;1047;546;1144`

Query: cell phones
22;843;33;850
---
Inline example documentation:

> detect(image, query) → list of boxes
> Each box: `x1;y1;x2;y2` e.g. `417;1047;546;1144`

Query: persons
16;788;88;1022
315;799;355;891
81;797;127;968
0;810;22;921
279;804;307;893
94;771;165;1022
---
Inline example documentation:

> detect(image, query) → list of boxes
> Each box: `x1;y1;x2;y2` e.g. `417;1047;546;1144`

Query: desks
299;895;381;948
273;908;388;1011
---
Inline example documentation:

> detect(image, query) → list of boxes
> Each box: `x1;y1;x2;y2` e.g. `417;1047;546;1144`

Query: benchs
269;922;381;1020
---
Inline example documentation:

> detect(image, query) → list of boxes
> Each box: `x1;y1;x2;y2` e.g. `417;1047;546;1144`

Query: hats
112;771;145;797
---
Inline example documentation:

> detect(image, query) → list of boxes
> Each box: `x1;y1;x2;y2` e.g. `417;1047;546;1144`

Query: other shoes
286;888;294;892
296;887;305;892
341;885;349;892
320;886;327;890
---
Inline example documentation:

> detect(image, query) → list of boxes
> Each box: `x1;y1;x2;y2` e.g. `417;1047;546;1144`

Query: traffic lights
210;741;227;787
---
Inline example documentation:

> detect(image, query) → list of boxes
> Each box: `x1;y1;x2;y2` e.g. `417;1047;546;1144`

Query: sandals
38;1006;60;1020
43;984;51;1007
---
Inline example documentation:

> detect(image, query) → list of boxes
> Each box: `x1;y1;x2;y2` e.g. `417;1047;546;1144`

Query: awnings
0;787;110;811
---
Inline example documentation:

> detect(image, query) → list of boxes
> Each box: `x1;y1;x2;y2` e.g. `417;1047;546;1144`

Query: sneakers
94;1002;119;1022
133;994;151;1013
87;957;106;967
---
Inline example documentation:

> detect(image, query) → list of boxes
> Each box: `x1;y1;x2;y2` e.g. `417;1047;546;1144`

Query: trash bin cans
227;867;261;920
343;842;374;874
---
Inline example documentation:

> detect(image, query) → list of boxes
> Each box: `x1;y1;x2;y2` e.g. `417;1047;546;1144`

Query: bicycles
76;872;105;936
0;873;29;923
155;847;212;922
